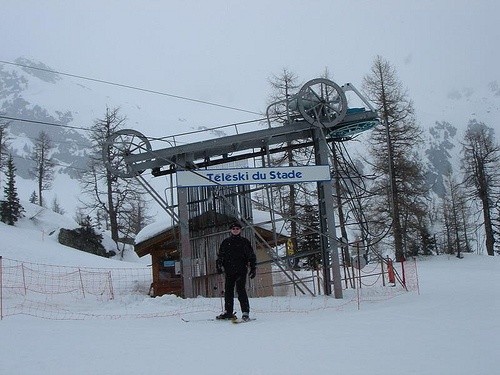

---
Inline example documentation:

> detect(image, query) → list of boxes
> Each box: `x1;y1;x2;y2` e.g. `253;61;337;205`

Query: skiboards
181;315;257;324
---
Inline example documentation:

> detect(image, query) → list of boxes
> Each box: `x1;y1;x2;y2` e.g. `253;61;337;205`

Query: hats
230;223;242;229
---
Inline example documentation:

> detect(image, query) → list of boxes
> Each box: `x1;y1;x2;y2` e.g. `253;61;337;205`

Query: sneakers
242;312;249;320
220;312;236;319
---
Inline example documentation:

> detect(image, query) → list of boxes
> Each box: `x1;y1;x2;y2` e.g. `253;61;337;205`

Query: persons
216;221;256;321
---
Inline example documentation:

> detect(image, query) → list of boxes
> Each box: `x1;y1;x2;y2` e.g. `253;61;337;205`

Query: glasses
232;228;239;230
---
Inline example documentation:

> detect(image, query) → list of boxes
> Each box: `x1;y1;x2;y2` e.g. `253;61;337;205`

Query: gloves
216;266;223;274
248;267;256;279
